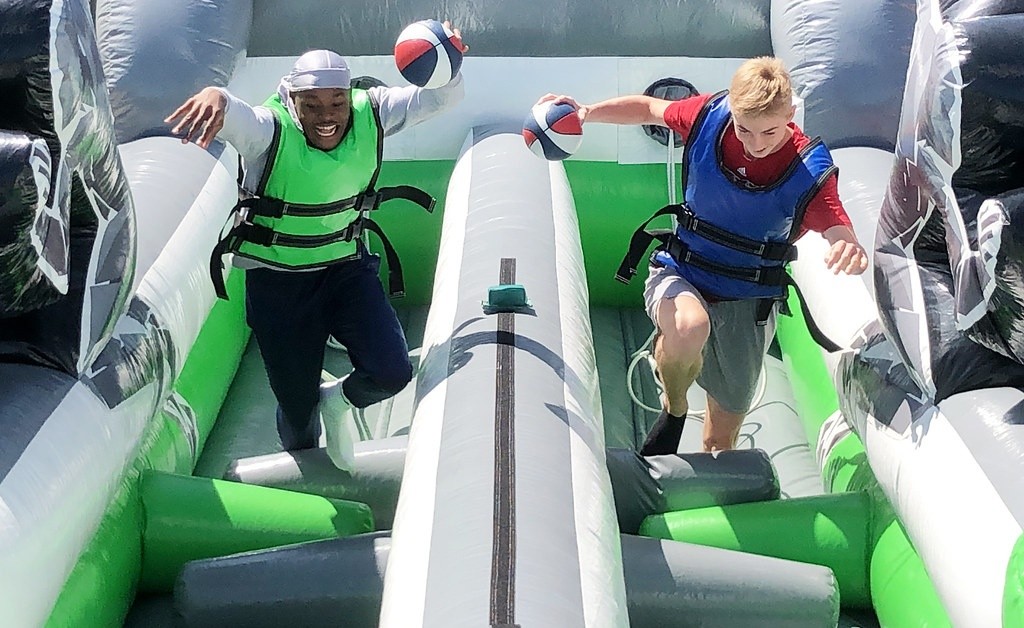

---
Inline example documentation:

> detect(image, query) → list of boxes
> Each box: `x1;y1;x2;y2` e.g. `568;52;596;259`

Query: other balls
522;102;584;164
393;19;464;89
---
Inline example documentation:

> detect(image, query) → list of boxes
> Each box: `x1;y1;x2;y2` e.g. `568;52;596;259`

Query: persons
164;16;469;469
539;55;869;457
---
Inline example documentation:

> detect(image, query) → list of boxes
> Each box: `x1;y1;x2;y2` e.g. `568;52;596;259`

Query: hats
289;50;351;92
